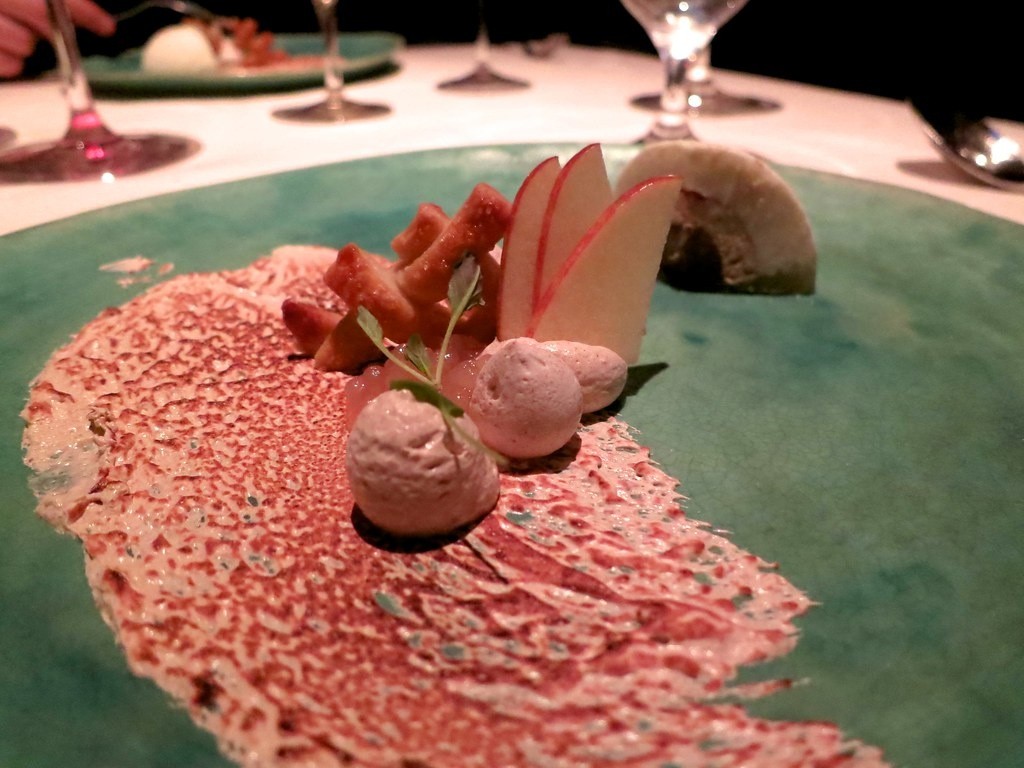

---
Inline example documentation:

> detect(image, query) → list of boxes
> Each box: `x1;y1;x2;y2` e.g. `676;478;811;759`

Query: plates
0;143;1024;767
83;31;405;96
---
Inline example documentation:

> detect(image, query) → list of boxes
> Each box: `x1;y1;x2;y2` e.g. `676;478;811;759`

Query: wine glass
437;0;531;92
618;0;781;144
273;0;391;124
0;1;201;184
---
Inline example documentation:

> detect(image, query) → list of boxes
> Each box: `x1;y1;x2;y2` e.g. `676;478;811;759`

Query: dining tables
0;46;1022;768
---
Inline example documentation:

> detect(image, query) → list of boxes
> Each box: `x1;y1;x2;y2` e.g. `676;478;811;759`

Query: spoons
907;96;1024;195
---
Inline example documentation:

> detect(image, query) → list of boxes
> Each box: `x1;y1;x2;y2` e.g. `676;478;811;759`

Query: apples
494;143;684;367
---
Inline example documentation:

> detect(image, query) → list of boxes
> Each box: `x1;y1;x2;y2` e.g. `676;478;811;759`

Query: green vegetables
355;255;512;465
